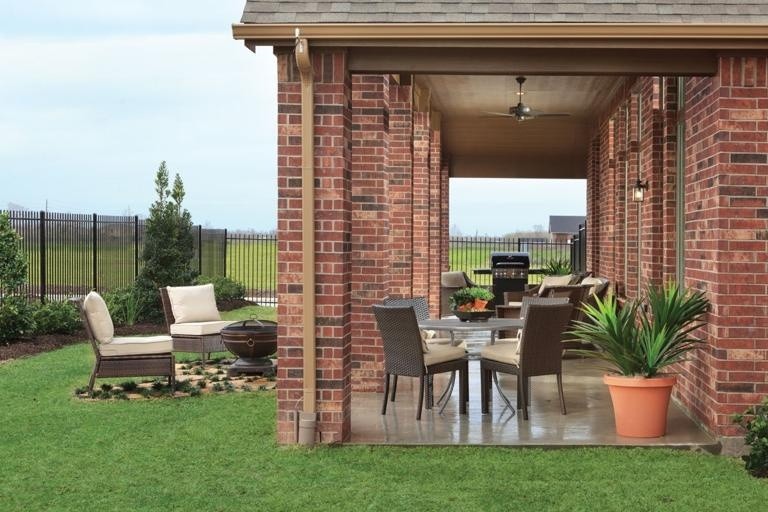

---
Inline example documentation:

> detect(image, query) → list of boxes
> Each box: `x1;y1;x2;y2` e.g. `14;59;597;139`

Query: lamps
632;176;648;202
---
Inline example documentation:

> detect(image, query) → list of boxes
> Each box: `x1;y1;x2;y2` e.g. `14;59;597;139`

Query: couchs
463;272;539;290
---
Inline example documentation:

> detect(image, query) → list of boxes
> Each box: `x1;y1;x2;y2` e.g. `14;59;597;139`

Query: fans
476;77;571;122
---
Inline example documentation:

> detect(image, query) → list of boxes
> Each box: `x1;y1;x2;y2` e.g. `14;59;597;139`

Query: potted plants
560;273;710;438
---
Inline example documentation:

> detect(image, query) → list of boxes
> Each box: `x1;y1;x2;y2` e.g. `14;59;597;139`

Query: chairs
440;272;468;318
481;304;573;420
383;297;469;408
495;284;594;339
371;304;469;420
73;291;175;392
485;296;570;343
503;274;580;305
561;277;610;358
158;284;238;367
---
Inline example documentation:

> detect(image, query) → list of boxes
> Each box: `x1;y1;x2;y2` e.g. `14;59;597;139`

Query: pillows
166;283;221;324
83;291;114;344
99;336;174;356
170;321;238;336
